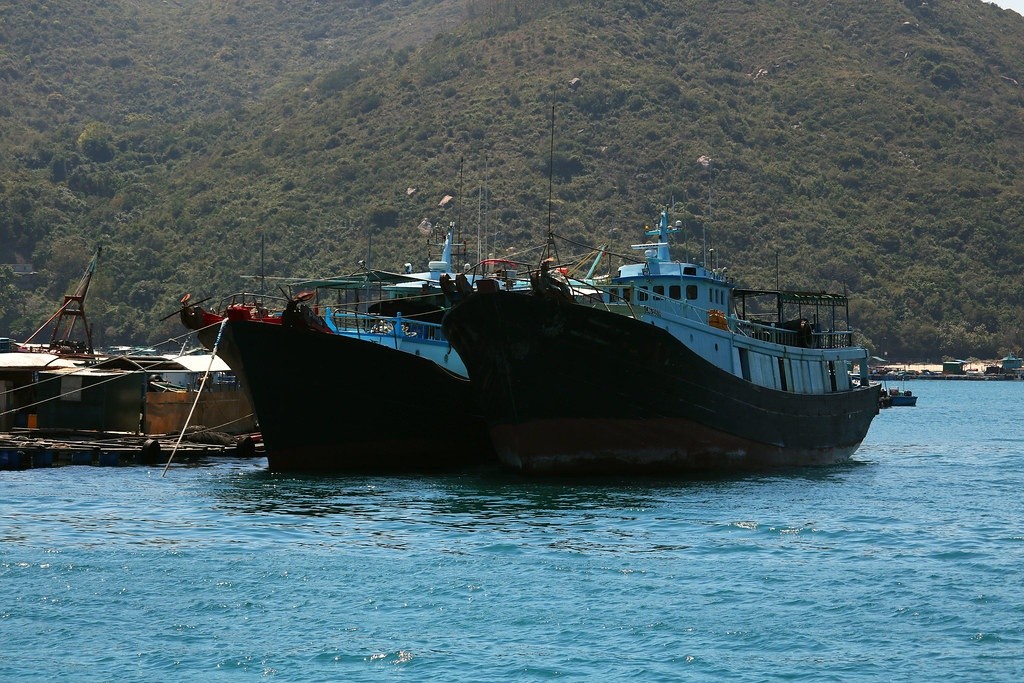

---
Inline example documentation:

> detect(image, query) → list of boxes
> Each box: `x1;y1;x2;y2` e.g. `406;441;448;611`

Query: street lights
880;337;887;359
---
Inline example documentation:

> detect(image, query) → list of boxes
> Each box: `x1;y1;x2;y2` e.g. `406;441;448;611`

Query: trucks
981;364;1004;375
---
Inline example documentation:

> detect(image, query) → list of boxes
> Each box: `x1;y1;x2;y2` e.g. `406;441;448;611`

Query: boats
890;391;918;406
439;204;882;472
180;221;609;477
